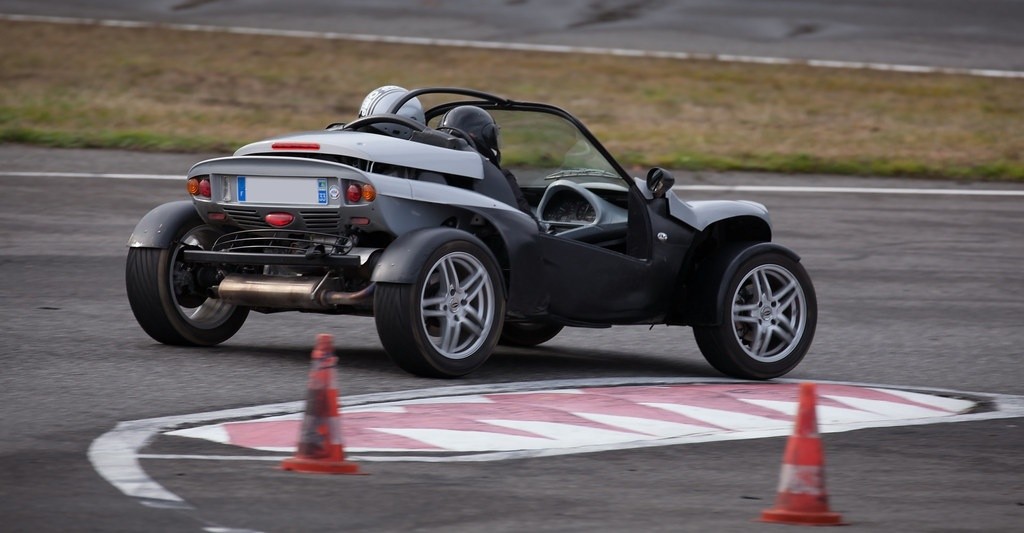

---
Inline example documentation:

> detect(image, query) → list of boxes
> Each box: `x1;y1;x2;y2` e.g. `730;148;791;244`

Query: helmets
357;85;426;126
437;105;502;161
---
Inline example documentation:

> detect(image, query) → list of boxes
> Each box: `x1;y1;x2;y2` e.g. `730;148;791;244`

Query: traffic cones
754;381;852;527
276;335;366;475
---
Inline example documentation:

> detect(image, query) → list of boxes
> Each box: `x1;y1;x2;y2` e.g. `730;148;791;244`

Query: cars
125;86;819;381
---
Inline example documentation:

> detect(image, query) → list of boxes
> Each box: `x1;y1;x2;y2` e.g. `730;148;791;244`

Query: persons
438;105;543;231
358;85;427;127
